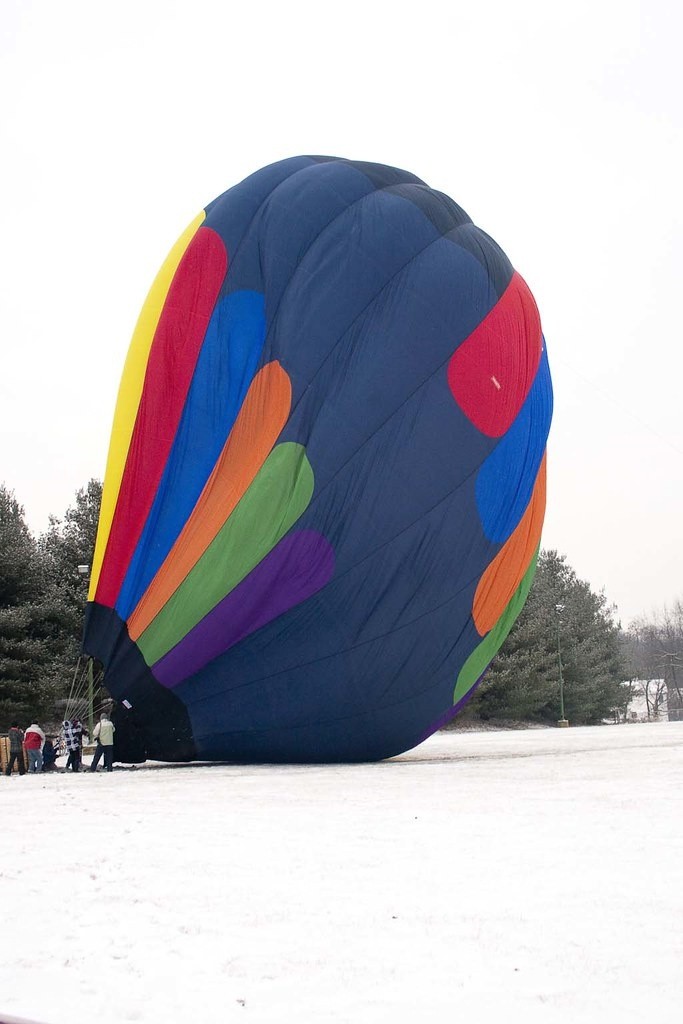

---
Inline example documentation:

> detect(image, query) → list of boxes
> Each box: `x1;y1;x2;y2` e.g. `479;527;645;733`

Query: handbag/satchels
94;737;99;742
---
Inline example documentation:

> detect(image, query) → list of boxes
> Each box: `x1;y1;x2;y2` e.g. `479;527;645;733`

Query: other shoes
87;768;93;773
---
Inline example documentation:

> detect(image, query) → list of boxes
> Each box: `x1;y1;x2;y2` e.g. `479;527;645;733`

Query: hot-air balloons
1;153;551;771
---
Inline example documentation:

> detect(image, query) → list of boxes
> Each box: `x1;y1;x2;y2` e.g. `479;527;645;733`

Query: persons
88;713;116;771
5;722;27;776
63;720;82;772
24;719;45;774
42;741;58;771
66;721;89;771
